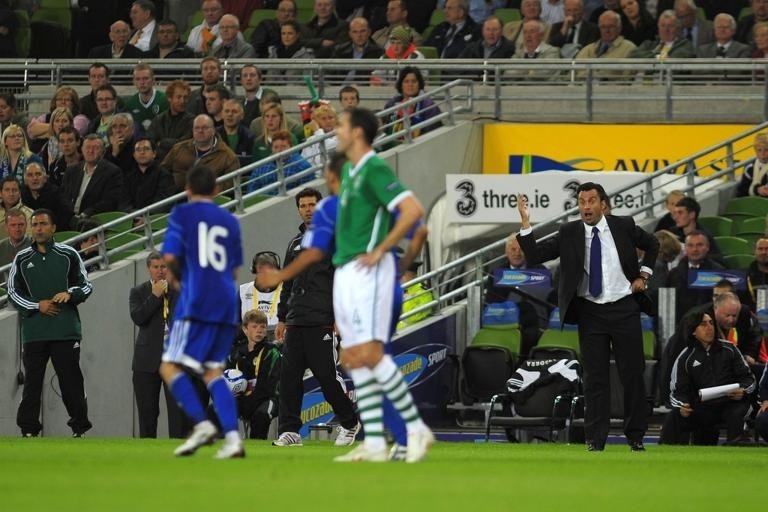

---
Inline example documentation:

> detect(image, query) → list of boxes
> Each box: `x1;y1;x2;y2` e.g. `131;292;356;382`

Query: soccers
224;369;247;397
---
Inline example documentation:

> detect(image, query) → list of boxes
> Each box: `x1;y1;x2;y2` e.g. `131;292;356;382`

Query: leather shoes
626;440;645;452
588;444;604;451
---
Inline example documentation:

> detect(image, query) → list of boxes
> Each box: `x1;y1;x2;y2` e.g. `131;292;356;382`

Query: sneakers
272;432;303;447
333;421;434;463
22;433;38;438
174;420;218;455
214;430;245;458
71;432;86;439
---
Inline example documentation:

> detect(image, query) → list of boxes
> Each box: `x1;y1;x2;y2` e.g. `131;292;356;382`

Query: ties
589;228;601;299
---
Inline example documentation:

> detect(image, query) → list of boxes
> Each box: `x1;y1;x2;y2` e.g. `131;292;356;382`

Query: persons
156;165;245;460
264;186;362;445
328;107;437;465
0;0;766;83
6;210;93;437
488;134;767;453
130;252;189;438
0;62;444;313
209;251;437;439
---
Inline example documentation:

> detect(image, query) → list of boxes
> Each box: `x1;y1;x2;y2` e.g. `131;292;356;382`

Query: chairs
0;4;532;79
395;186;765;412
52;190;276;267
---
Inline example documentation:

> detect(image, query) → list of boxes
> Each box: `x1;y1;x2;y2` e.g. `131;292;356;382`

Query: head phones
249;250;280;273
